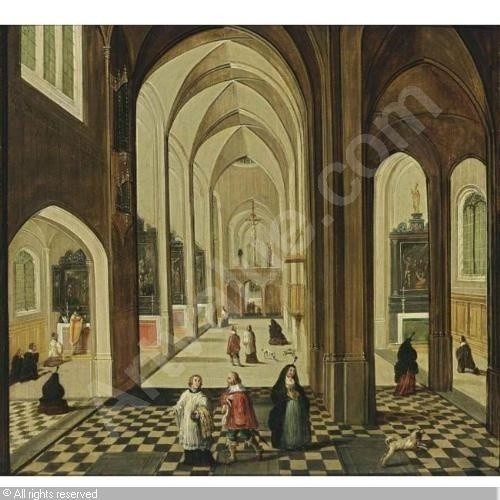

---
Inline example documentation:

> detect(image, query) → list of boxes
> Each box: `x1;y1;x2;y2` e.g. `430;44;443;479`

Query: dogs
282;347;296;359
260;347;275;361
380;428;429;469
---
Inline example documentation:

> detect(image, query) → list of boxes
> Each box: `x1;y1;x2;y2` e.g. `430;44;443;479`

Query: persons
39;373;65;405
246;301;263;316
268;318;287;344
70;312;84;355
10;343;40;382
218;370;264;464
455;334;480;375
243;324;259;363
226;325;245;367
414;271;426;289
392;339;417;396
269;364;311;454
169;375;217;471
401;253;412;288
48;332;62;357
